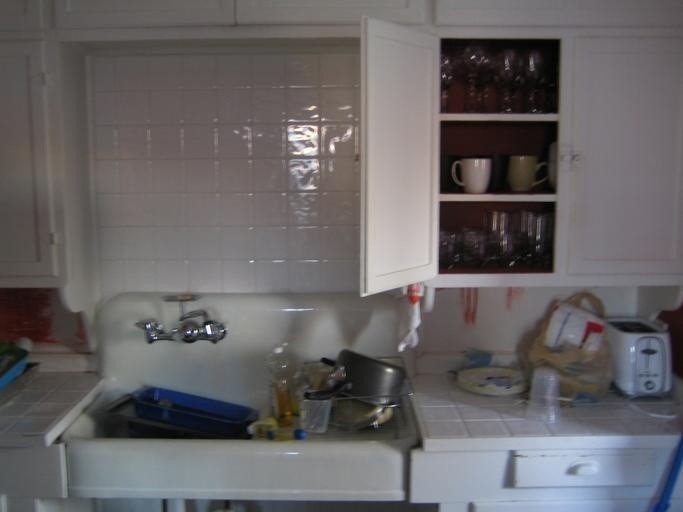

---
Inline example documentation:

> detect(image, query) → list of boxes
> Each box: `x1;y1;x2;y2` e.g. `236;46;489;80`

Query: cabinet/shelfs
1;35;97;290
355;17;683;300
407;443;683;510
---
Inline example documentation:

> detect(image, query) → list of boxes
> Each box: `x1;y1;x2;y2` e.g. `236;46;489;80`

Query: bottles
270;346;294;428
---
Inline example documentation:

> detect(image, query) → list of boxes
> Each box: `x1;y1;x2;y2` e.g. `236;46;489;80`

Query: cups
507;155;551;194
525;368;562;425
451;156;493;194
438;208;550;274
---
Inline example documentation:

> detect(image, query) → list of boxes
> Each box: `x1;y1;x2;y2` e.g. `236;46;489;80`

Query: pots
301;346;415;412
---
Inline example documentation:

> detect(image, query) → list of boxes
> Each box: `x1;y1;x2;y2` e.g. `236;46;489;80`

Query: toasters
603;316;673;400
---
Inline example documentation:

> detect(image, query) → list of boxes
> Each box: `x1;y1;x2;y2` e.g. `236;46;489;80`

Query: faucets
134;308;226;343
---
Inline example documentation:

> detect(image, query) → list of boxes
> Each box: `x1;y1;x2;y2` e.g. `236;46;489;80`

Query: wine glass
441;36;555;114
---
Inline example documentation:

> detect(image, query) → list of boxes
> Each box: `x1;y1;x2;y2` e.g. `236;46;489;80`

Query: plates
457;366;529;396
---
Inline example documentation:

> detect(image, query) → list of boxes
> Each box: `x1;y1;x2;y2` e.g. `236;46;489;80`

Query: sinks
60;289;419;502
